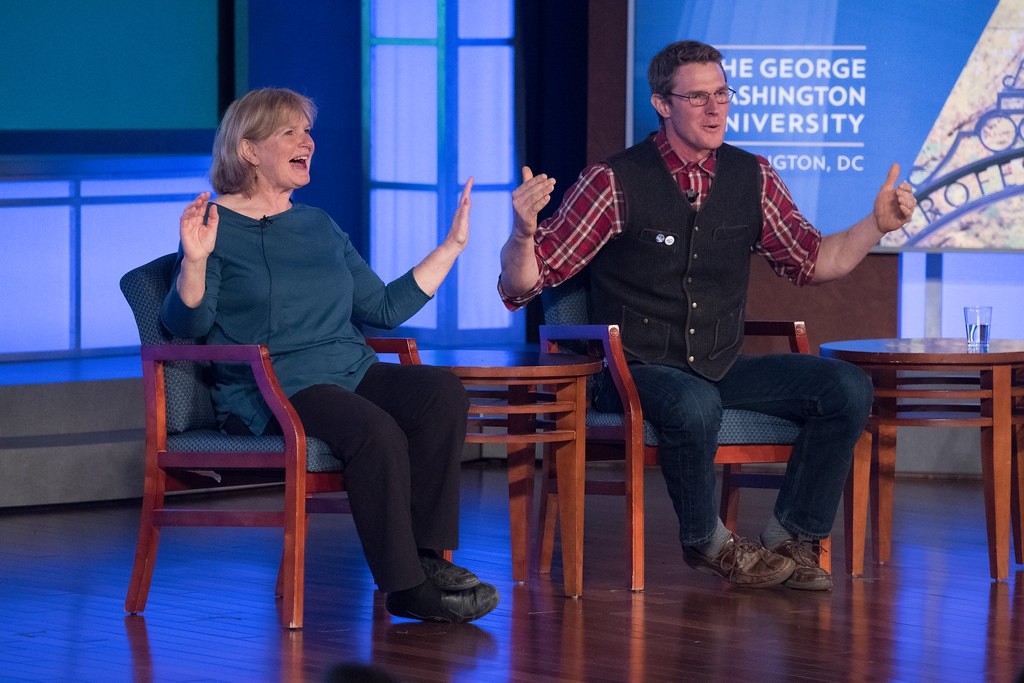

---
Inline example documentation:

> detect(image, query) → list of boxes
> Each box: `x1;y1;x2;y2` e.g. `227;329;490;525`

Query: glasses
662;86;737;106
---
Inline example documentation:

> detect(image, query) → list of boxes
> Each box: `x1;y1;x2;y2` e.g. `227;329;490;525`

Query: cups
963;305;993;346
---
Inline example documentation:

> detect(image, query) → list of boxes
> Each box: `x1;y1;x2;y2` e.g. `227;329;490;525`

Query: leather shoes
683;531;796;587
387;581;498;622
419;553;480;589
758;531;834;589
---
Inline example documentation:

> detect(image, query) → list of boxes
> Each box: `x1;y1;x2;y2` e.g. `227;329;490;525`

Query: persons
157;86;499;624
497;39;918;590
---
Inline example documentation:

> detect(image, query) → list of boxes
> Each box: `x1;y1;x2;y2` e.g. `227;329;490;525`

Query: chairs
116;251;421;629
531;271;831;590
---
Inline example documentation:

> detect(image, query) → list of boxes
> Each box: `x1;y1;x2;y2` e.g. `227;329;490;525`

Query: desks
820;337;1024;579
375;350;603;598
